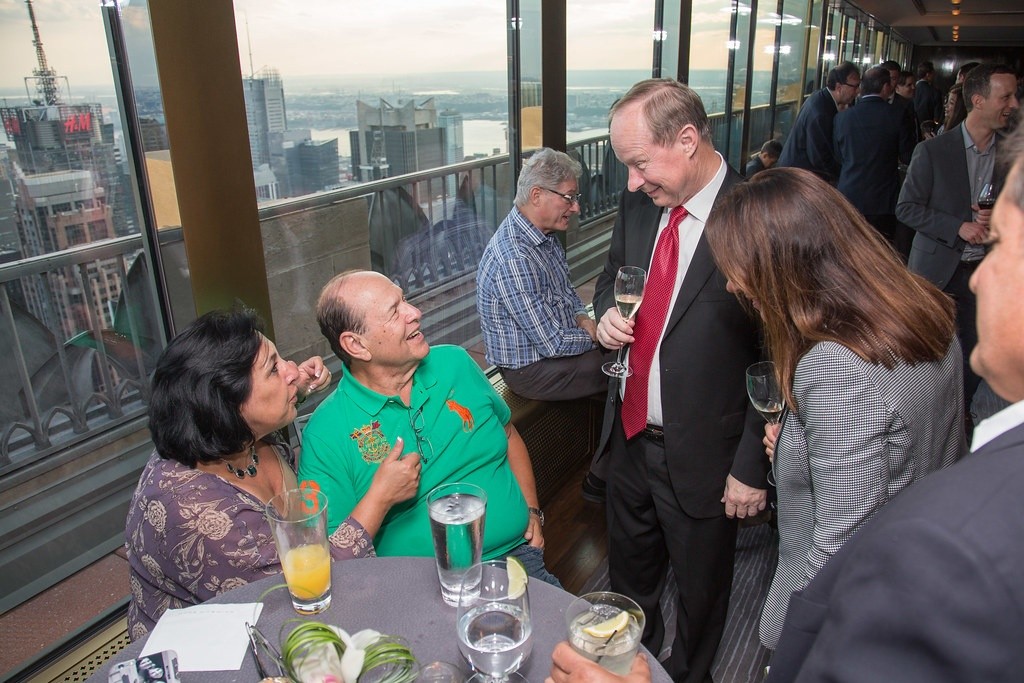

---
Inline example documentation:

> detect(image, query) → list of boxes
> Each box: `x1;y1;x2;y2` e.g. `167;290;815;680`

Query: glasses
539;187;582;206
843;83;860;90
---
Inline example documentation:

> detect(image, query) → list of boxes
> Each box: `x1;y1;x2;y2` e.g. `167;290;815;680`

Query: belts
640;428;664;441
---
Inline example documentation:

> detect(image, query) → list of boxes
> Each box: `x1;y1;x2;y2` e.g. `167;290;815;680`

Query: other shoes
581;472;607;504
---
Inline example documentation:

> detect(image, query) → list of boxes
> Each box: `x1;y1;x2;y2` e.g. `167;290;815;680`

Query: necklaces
225;446;259;478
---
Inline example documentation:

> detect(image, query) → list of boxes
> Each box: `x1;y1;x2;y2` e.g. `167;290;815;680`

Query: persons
124;309;422;643
895;71;915;100
914;62;944;129
832;66;918;264
924;87;968;140
592;78;771;683
776;61;861;182
955;63;980;85
706;166;968;648
896;61;1020;447
476;148;619;505
556;154;1024;683
299;270;563;590
388;161;494;296
746;139;783;177
881;60;918;135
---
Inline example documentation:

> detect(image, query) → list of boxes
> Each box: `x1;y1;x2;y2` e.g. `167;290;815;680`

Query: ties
621;205;690;440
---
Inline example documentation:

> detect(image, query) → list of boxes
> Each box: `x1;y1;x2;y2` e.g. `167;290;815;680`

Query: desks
83;557;672;683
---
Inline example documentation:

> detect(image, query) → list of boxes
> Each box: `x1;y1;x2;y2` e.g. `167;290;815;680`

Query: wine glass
745;361;790;486
455;560;534;683
601;266;646;377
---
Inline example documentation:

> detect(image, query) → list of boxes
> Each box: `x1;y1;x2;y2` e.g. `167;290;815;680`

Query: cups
977;184;1000;211
265;487;332;615
426;483;488;606
564;591;646;677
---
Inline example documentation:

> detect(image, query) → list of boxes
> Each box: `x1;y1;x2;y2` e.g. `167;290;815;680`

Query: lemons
581;611;632;636
507;556;529;600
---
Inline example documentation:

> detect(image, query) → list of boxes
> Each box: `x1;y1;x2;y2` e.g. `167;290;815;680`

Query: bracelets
529;507;545;526
